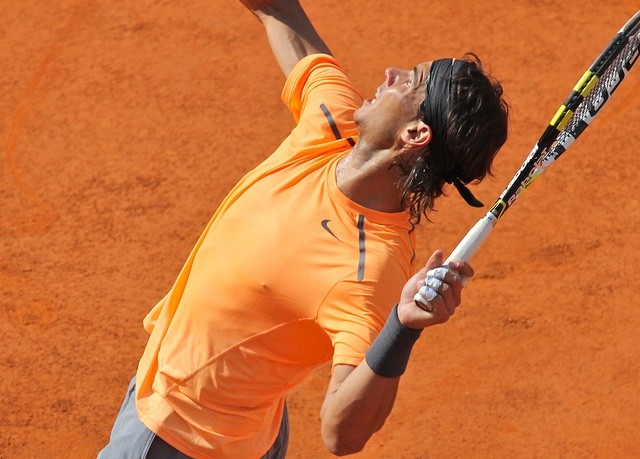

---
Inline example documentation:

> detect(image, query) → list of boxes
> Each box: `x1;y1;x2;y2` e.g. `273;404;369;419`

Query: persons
95;1;509;459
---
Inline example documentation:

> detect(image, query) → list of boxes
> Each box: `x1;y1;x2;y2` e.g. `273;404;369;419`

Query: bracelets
365;304;422;379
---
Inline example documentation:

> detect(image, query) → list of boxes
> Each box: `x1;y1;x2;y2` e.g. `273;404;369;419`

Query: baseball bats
414;10;640;312
